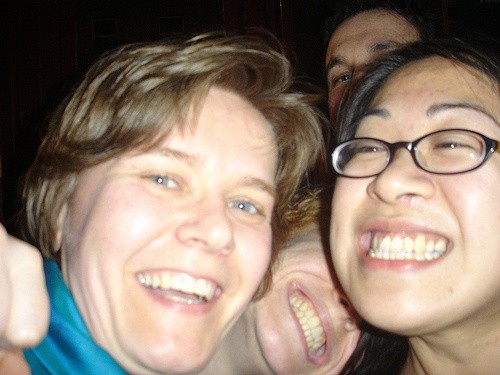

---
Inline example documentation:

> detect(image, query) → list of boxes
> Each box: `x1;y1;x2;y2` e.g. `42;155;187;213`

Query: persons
330;39;500;375
196;187;410;375
324;4;433;151
0;31;324;375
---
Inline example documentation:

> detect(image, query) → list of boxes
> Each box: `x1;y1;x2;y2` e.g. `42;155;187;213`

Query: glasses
331;128;500;178
